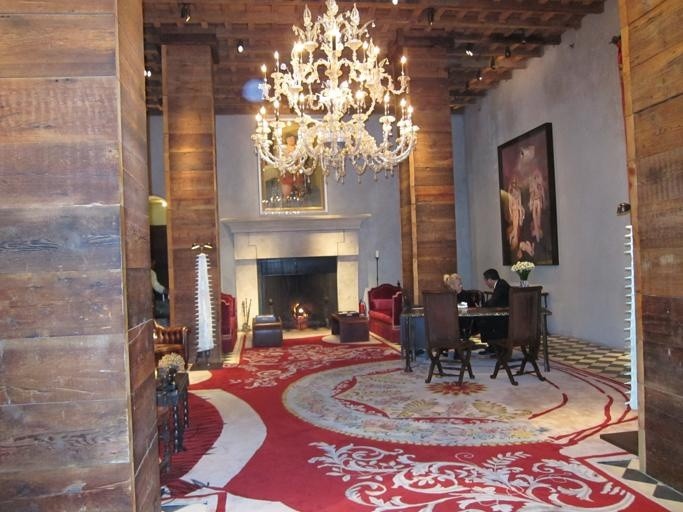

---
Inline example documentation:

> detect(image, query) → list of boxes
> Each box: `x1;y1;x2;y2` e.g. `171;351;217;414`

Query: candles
374;250;380;257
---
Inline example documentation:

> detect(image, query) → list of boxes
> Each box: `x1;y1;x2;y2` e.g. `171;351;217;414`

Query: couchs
368;282;404;343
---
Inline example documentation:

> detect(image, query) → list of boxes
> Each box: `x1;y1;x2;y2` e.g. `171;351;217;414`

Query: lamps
248;0;418;187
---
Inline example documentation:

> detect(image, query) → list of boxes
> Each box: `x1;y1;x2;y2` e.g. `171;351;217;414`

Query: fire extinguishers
359;299;366;316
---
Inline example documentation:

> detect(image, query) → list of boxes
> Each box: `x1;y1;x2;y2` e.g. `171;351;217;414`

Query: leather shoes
479;345;512;358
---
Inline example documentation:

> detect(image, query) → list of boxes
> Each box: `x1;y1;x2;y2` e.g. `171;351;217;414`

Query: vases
518;278;528;287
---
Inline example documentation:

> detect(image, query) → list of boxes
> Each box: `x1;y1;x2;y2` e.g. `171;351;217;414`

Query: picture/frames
257;121;327;217
496;119;562;266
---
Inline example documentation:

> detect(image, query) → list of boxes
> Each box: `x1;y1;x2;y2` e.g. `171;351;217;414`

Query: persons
440;273;474;356
276;134;308;198
506;174;526;249
528;166;546;243
477;268;511;356
150;255;168;295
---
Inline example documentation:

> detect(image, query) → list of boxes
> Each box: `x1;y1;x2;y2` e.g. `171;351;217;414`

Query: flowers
510;260;533;278
157;347;185;372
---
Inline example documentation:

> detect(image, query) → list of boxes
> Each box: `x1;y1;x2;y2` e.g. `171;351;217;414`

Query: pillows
371;298;395;312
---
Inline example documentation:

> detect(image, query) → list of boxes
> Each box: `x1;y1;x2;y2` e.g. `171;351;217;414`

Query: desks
156;370;191;457
400;307;554;375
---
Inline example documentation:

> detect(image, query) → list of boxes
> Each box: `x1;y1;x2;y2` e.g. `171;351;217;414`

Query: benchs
220;292;236;351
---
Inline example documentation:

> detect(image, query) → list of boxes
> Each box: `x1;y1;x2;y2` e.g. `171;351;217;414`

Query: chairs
421;287;477;385
490;284;544;387
153;320;188;371
